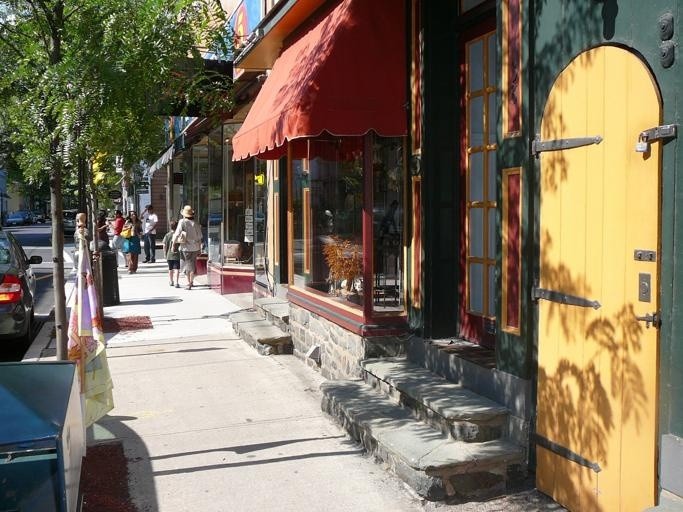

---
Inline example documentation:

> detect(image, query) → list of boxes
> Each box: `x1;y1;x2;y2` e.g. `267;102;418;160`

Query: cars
61;208;78;235
6;210;47;226
1;231;42;346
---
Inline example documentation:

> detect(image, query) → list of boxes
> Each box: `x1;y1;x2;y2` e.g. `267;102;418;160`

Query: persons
140;204;158;263
121;211;142;274
161;220;182;289
171;204;203;290
108;210;129;268
97;209;110;244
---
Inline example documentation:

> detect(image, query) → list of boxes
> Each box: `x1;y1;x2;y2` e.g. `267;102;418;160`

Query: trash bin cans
89;240;120;307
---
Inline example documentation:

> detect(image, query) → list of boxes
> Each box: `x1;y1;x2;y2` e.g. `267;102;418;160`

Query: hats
180;205;196;218
145;204;153;208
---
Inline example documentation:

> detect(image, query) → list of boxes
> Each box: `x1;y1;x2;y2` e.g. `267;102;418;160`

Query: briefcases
193;252;209;276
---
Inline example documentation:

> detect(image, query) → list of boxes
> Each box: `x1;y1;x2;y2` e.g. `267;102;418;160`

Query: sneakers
169;281;193;290
128;269;136;274
142;258;155;263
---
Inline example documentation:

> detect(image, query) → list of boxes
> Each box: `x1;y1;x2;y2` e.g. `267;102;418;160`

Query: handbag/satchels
171;231;187;244
120;226;132;237
